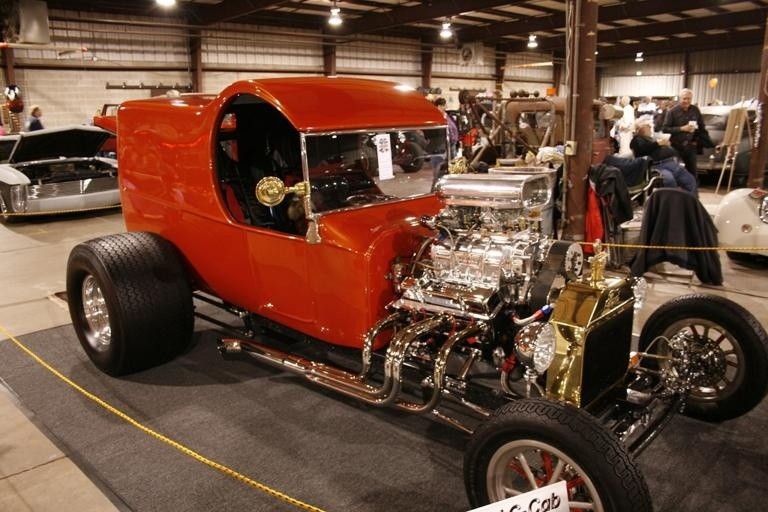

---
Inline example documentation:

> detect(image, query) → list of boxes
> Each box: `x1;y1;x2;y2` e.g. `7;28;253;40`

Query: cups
688;121;697;133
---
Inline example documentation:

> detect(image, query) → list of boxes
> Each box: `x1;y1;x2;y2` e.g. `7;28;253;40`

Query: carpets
1;320;768;507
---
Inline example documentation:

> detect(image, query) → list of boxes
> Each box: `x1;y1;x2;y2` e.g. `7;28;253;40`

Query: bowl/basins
655;132;671;141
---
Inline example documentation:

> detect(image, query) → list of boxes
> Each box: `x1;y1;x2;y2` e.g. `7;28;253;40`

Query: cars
0;122;125;222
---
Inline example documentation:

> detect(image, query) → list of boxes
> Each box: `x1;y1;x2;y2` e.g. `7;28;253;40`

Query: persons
434;86;720;208
28;107;45;132
